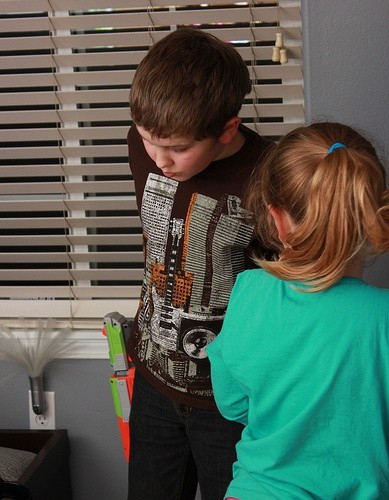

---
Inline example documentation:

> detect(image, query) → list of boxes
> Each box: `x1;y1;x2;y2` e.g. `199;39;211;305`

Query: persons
125;31;368;500
204;120;389;500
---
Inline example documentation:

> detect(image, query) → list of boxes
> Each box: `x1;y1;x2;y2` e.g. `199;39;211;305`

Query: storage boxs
0;428;74;500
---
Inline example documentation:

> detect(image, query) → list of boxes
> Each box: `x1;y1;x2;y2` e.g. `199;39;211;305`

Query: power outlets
28;390;56;430
